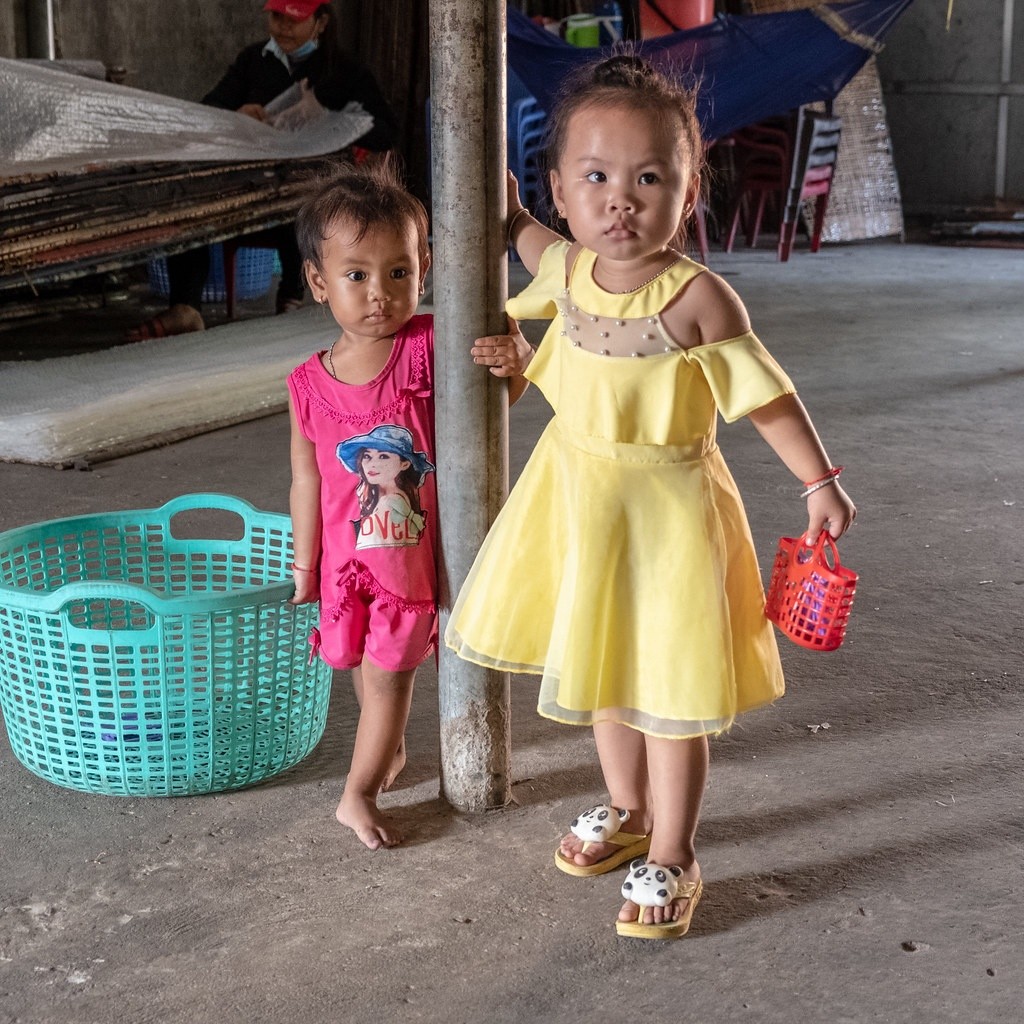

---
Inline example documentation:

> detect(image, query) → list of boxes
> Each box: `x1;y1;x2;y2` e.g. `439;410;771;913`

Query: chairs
424;64;552;263
691;100;843;269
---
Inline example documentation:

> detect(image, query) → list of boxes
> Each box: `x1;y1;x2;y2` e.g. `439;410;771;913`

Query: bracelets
290;562;318;574
803;467;841;487
800;475;840;498
509;208;529;247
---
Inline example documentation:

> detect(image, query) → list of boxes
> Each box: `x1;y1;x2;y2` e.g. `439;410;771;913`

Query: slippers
554;805;652;877
616;859;703;939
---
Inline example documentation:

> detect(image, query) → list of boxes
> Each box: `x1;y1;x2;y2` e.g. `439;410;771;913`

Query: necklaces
616;256;683;294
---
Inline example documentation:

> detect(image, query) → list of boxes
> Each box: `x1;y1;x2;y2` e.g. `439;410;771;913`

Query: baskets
0;492;333;798
148;242;275;301
764;529;860;652
273;251;283;278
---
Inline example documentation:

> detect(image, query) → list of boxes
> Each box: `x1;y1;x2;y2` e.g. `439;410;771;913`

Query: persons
123;0;390;337
433;53;860;942
283;174;545;854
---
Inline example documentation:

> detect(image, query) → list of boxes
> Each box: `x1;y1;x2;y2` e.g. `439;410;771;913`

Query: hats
263;0;332;21
